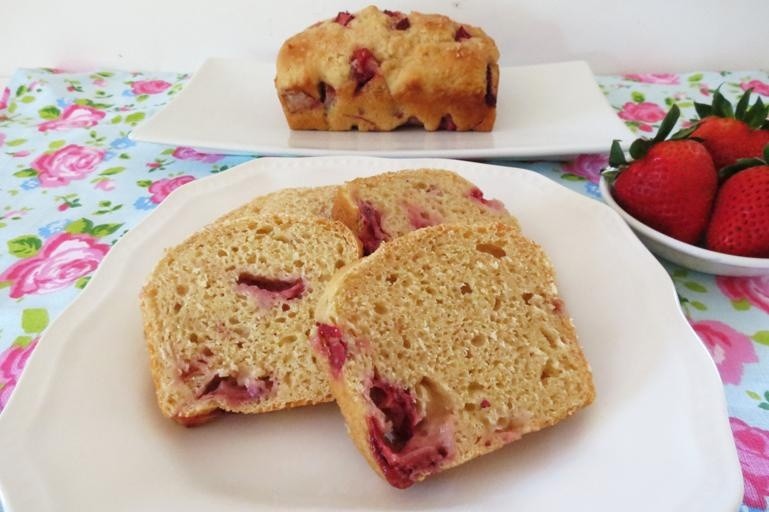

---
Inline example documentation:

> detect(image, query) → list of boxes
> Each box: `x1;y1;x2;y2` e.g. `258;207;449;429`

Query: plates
599;156;769;285
128;52;638;155
0;156;745;512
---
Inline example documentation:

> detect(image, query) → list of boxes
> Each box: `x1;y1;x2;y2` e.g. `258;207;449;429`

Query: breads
332;168;511;257
274;5;499;131
214;185;366;240
140;214;364;429
309;223;594;489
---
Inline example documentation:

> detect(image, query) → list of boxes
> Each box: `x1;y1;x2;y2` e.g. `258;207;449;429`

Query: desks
0;69;768;512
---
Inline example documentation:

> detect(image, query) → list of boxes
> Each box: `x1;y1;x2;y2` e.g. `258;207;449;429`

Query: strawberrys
601;87;769;258
